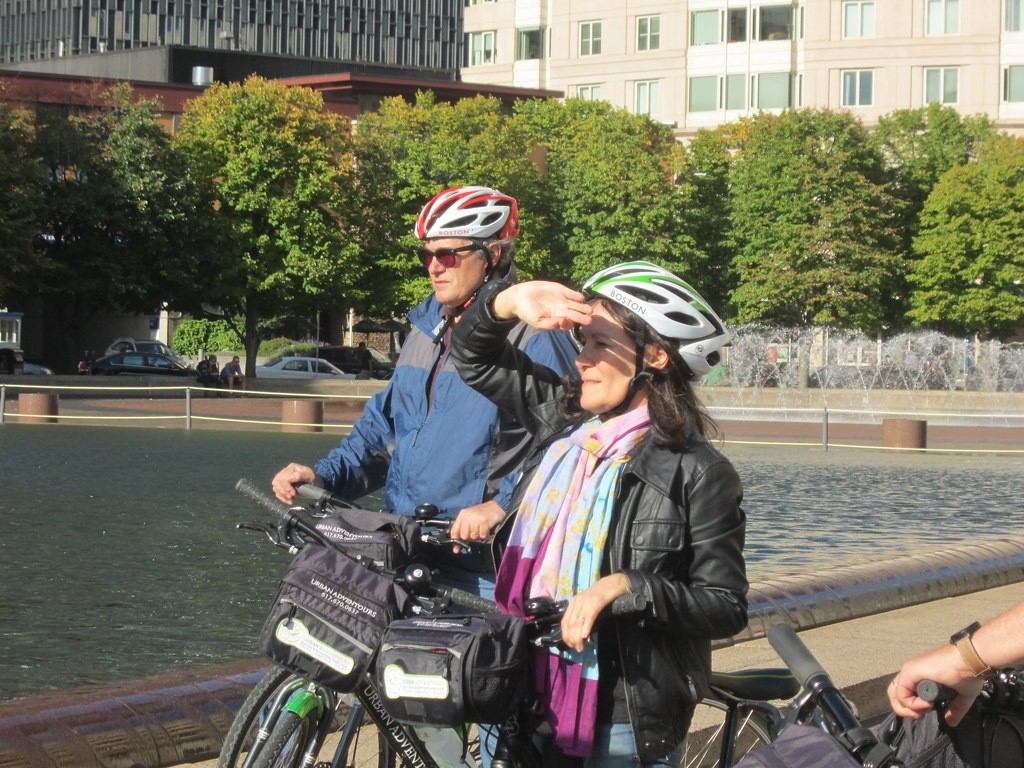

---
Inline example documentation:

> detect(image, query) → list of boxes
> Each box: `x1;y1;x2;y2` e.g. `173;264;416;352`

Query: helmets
415;186;519;246
582;260;732;380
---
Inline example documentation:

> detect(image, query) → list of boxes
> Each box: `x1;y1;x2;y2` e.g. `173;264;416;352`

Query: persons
220;356;247;397
923;337;953;390
354;343;373;377
197;355;223;398
888;600;1024;726
272;185;581;768
451;263;749;768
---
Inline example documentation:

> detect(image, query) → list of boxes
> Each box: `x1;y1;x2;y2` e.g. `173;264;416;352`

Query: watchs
951;621;1001;680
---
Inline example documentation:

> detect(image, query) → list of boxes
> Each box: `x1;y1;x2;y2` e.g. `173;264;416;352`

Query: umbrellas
342;318;413;360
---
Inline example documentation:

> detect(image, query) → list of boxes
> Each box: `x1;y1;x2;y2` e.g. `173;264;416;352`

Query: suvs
105;336;175;366
298;345;395;380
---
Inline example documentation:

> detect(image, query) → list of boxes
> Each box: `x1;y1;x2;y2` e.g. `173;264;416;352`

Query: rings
577;615;585;620
892;679;898;687
470;533;479;535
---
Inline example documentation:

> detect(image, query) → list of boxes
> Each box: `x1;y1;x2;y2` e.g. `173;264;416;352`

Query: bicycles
234;477;1024;768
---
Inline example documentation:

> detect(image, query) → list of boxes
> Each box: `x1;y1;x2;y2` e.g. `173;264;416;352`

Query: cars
88;351;199;376
255;356;378;380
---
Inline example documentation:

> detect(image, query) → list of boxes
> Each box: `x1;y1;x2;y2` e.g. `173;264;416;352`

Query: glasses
416;243;480;268
